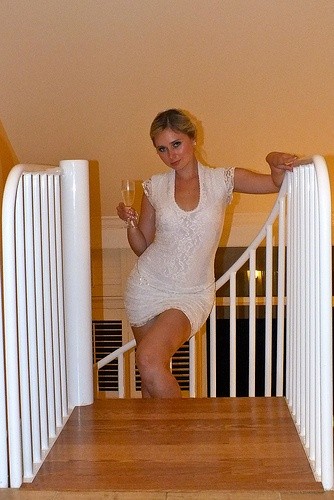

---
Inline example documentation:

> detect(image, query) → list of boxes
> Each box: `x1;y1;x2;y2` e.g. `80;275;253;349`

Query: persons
116;106;303;400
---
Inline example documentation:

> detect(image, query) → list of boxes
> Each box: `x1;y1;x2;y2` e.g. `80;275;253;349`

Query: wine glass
120;177;136;230
